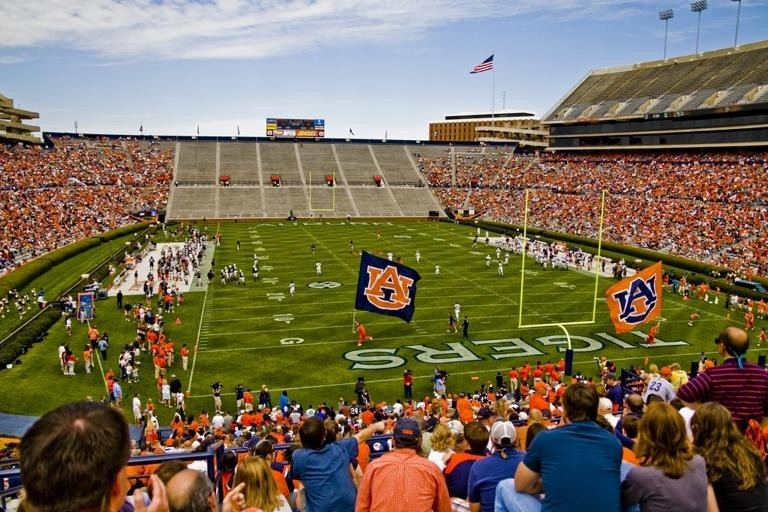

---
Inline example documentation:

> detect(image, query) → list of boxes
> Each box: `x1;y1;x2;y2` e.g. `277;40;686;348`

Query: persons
1;133;768;512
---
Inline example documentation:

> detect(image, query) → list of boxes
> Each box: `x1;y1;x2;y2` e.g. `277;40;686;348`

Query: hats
394;418;419;444
599;398;612;410
660;368;672;374
491;418;517;446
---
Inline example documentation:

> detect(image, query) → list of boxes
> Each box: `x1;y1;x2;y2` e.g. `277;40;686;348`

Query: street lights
690;1;708;56
658;9;673;58
730;0;742;45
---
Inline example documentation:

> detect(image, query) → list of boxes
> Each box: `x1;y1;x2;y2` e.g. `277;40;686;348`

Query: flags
467;55;493;74
